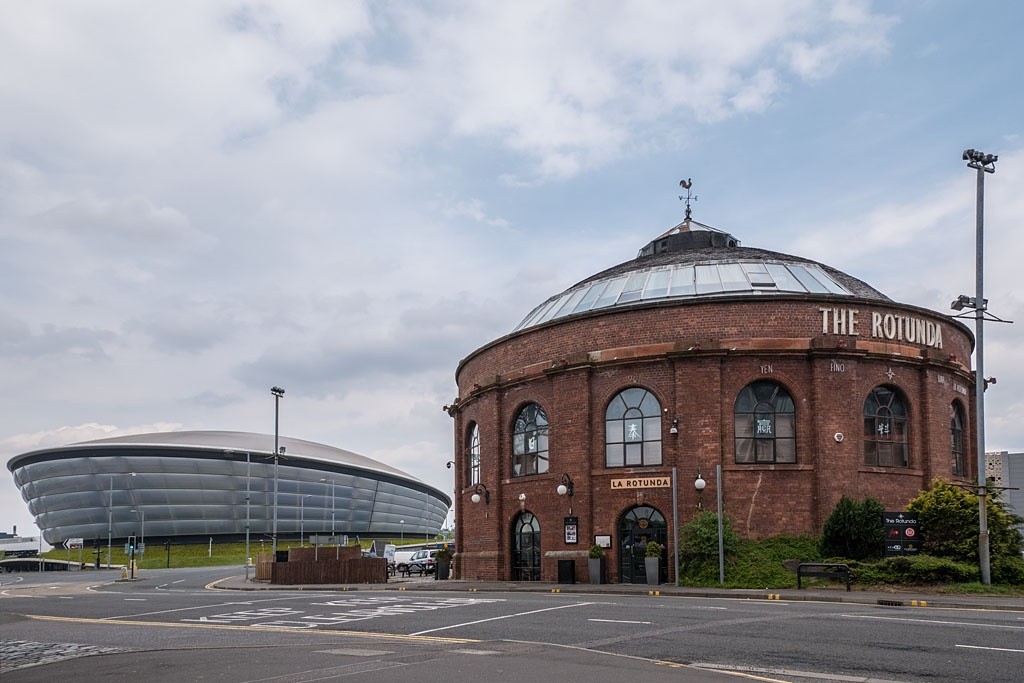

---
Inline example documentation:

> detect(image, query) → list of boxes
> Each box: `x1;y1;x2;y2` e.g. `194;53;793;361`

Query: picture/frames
594;535;612;549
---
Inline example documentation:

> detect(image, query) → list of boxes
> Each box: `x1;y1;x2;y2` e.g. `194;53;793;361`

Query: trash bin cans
437;560;450;580
558;560;575;584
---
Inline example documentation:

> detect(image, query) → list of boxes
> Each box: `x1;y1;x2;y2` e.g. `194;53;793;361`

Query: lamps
693;467;706;493
472;484;489;505
557;473;574;497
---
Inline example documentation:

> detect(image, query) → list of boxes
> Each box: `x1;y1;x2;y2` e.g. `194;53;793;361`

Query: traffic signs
63;538;84;551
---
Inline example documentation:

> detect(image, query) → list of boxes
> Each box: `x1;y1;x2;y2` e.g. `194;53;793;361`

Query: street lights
446;509;454;529
400;520;405;545
417;492;429;549
224;449;249;579
944;148;1014;588
320;478;335;547
300;494;313;548
264;386;290;563
130;509;145;561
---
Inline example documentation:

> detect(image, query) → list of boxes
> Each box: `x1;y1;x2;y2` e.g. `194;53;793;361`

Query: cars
404;548;447;574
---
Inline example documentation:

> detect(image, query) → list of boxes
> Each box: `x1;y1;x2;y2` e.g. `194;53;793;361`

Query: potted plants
588;544;606;585
645;541;662;585
434;548;453;580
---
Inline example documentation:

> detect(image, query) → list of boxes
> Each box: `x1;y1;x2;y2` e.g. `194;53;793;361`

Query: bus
393;542;456;572
